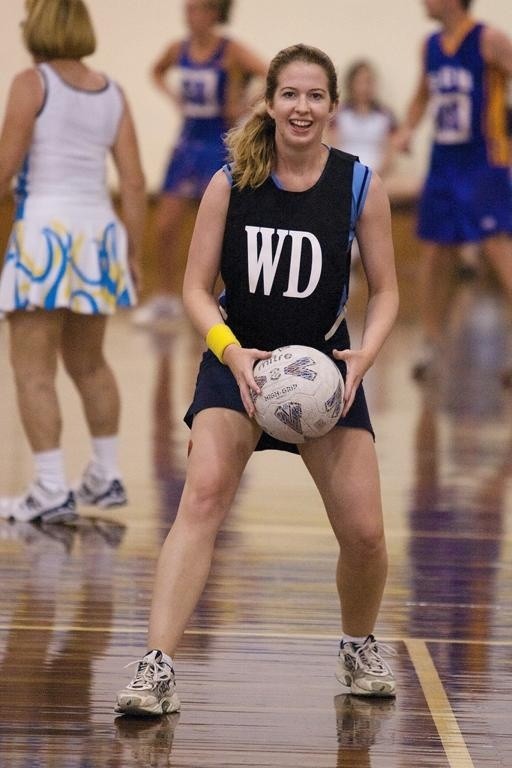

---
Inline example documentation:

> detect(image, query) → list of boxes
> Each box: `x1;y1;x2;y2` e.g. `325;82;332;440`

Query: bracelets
206;326;241;367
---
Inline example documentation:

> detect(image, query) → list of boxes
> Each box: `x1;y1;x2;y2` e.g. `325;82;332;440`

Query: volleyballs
250;344;345;444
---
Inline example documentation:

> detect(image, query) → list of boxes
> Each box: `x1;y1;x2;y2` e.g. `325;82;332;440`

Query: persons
113;44;406;718
1;0;146;525
133;0;269;336
321;61;412;294
379;1;512;385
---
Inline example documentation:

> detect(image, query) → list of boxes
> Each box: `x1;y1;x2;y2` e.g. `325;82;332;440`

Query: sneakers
113;650;180;718
75;475;128;510
1;478;79;526
334;634;397;699
